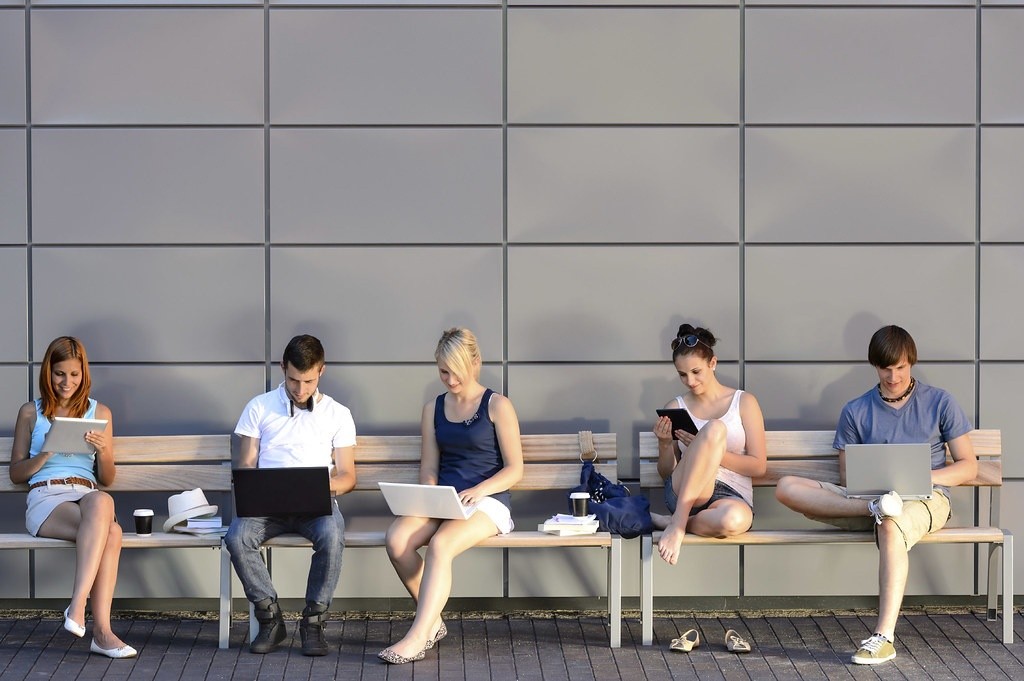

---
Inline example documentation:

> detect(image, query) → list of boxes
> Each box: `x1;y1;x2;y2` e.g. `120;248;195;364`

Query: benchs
639;429;1012;647
247;430;621;649
0;435;233;649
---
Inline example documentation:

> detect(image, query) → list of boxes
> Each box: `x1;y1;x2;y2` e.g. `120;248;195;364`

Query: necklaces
877;376;915;402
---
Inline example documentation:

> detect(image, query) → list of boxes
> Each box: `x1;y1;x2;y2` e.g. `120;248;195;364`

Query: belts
28;476;97;493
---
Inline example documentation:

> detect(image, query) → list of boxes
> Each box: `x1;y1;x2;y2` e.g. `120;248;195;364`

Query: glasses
671;335;710;350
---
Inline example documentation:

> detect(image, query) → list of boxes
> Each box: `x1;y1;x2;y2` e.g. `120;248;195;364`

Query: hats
163;488;218;532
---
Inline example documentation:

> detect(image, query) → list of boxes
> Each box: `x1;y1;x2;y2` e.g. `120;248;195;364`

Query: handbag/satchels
567;450;653;539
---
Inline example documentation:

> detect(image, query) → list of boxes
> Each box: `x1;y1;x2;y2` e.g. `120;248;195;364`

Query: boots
300;606;330;655
250;597;286;652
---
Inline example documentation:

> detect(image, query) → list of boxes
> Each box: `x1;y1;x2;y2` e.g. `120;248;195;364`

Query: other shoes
90;636;137;658
725;629;751;652
63;605;86;638
377;646;426;664
423;622;448;650
670;629;700;651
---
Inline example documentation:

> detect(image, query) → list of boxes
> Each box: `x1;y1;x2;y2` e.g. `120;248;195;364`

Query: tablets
656;408;699;441
41;417;110;454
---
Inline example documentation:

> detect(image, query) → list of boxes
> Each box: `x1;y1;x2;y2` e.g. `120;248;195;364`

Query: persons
378;329;526;661
10;335;138;657
223;334;357;656
649;323;768;564
775;324;978;664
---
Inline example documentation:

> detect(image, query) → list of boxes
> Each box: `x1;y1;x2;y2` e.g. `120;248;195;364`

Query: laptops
845;443;932;500
376;482;481;520
233;468;337;517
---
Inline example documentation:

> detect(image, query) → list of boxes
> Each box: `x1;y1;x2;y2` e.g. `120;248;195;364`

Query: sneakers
868;491;903;525
851;633;896;664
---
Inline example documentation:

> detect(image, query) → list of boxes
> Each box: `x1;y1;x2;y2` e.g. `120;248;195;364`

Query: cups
570;492;590;516
133;509;154;536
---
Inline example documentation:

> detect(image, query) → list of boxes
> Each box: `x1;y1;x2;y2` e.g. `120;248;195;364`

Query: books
174;517;229;538
538;513;599;536
